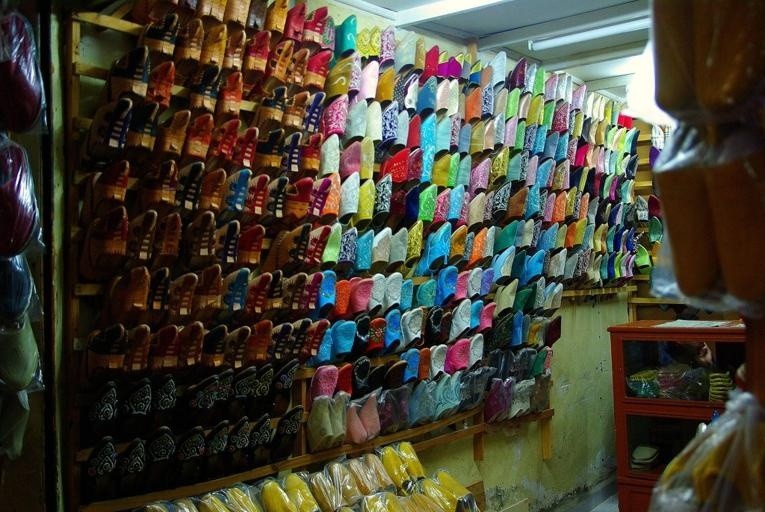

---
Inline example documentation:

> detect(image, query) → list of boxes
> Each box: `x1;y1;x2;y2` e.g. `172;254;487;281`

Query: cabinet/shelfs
605;318;747;512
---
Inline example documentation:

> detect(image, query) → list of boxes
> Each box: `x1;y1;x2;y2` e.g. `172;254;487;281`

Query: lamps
527;17;653;53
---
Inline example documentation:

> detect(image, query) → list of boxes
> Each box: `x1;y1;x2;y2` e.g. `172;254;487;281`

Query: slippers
649;146;661;166
636;195;647;222
648;195;663;219
640;268;653;275
88;404;304;485
1;254;35;324
104;266;321;323
315;267;482;320
141;13;332;92
1;389;31;460
2;314;40;393
487;176;638;289
91;160;333;227
320;223;490;280
88;99;324;178
487;49;642;176
316;133;494;199
311;299;495;364
145;441;480;512
637;244;652;266
307;362;500;454
331;12;483;84
487;276;564;423
308;333;486;399
2;12;46;133
83;206;332;275
651;241;662;266
90;319;331;370
0;141;39;258
89;359;299;425
110;48;327;133
321;93;487;159
651;127;665;149
326;58;484;122
161;0;337;52
649;217;665;245
321;172;487;235
